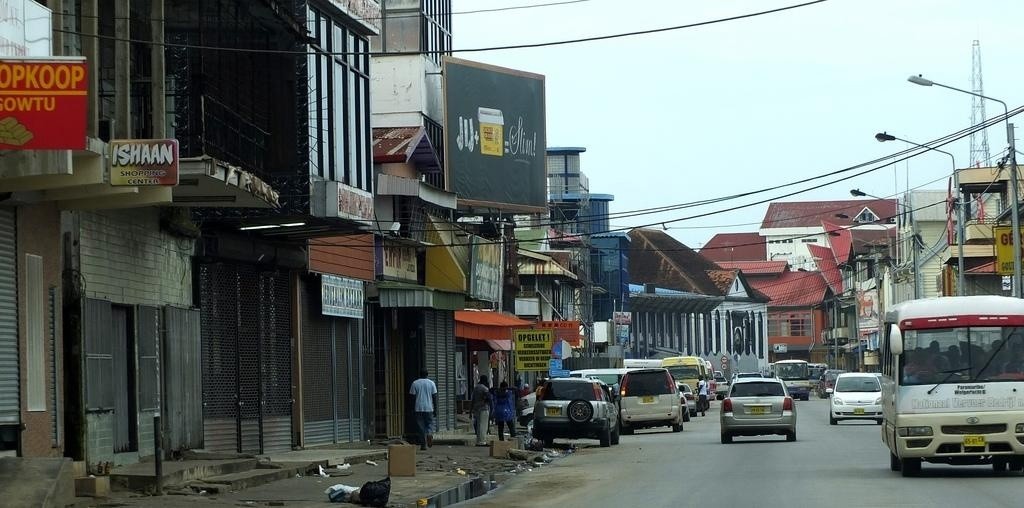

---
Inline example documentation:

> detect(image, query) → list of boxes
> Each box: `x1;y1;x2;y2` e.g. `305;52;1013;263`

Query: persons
410;368;441;447
469;372;548;448
904;336;1023;380
696;376;708;414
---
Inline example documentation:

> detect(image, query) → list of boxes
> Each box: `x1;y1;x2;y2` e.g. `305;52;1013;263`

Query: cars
734;373;764;378
676;389;691;422
714;370;722;377
730;373;736;383
720;378;796;441
677;383;697;417
829;373;883;425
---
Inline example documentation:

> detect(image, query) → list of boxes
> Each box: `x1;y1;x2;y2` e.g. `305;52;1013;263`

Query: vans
518;368;647;427
618;368;684;434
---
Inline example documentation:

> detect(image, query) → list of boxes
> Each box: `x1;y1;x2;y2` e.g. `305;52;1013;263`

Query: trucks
622;358;661;368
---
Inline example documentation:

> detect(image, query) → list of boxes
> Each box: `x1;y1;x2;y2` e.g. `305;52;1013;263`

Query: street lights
812;256;862;372
908;73;1022;298
849;188;917;298
874;131;965;299
798;267;838;368
834;212;896;304
828;232;882;362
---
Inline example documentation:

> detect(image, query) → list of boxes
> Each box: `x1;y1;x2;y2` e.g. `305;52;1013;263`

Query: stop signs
720;356;728;363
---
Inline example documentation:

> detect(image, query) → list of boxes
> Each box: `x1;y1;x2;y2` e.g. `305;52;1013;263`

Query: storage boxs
386;443;417;477
73;474;111;494
489;440;521;458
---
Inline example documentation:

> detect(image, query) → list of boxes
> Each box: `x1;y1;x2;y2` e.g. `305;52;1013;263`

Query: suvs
817;369;848;399
534;377;618;446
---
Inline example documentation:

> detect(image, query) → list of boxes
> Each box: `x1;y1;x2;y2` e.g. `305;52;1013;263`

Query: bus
773;360;809;400
767;363;828;391
882;294;1023;476
704;360;715;401
660;356;709;411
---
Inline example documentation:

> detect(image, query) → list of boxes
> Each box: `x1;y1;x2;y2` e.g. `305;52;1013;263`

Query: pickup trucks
715;377;729;398
